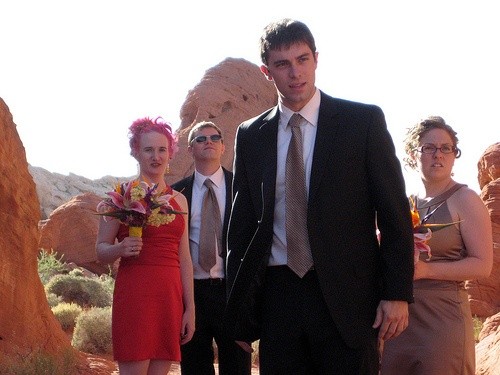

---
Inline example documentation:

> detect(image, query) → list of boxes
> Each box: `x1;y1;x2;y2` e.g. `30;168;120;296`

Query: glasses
413;144;453;153
189;135;223;146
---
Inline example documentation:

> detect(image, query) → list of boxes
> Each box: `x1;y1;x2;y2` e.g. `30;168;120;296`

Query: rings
131;246;134;252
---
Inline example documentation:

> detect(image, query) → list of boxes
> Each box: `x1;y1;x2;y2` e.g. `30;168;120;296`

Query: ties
284;113;314;279
198;178;224;272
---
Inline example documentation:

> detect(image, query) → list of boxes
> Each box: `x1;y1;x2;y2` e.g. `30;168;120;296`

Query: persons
97;117;195;375
171;122;253;375
222;19;415;375
376;116;493;375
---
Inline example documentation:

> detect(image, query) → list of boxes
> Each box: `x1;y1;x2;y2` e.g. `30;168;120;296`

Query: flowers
94;180;189;236
376;196;464;265
